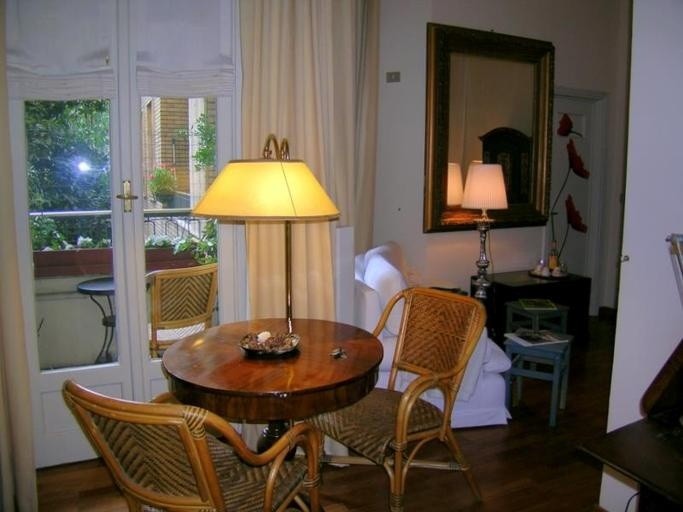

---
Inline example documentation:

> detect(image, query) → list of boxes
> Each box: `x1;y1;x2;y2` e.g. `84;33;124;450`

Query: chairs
304;286;488;511
354;241;513;430
61;377;322;512
145;265;218;359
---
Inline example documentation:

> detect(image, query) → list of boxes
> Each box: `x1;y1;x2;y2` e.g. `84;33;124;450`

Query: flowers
146;163;178;200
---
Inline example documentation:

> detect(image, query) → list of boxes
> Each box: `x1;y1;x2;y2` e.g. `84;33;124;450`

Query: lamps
446;161;464;212
461;163;509;300
188;133;352;460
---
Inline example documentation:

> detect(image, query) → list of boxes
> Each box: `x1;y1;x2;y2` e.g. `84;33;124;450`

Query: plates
238;331;300;355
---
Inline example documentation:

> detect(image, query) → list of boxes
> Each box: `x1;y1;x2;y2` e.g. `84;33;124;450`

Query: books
504;328;569;347
519;298;557;311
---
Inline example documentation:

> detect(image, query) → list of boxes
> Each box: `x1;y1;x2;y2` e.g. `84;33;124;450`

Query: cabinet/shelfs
469;268;593;361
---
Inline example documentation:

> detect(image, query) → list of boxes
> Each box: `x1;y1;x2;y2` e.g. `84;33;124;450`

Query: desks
75;277;150;366
582;414;683;511
160;320;384;464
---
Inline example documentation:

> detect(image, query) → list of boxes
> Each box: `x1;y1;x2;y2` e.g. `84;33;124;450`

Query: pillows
364;256;409;335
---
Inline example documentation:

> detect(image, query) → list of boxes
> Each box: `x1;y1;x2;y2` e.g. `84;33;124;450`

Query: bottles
549;240;559;272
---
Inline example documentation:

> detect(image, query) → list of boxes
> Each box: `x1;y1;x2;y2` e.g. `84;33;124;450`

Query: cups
534;265;561;277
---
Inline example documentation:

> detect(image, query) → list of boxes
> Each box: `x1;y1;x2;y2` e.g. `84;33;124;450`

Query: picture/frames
423;22;555;235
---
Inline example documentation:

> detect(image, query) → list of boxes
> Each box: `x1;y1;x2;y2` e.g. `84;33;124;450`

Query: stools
505;300;569;373
501;328;574;427
